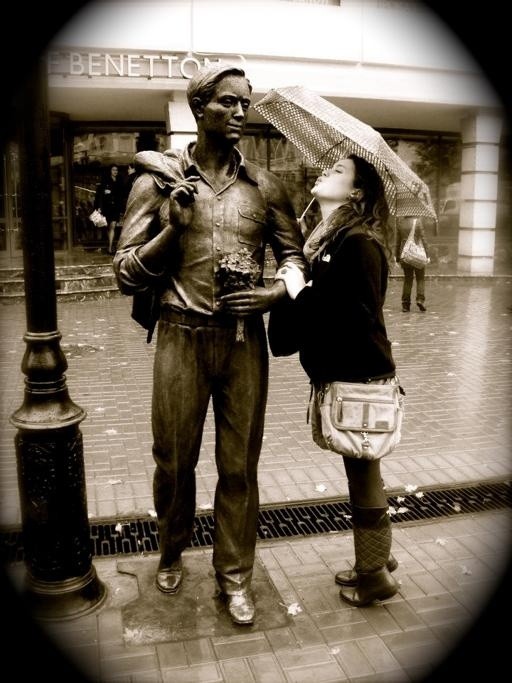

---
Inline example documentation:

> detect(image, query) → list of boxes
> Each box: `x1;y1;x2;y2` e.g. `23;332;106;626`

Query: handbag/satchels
400;219;428;270
311;382;403;460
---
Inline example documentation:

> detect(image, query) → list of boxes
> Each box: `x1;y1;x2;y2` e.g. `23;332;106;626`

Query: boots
336;505;400;607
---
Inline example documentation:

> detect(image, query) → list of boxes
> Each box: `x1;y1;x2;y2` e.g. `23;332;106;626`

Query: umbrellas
253;85;440;225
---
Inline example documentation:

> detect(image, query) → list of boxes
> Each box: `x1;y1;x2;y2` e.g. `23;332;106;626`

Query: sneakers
401;300;430;312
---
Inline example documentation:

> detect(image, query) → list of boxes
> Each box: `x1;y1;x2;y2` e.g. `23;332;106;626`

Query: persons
394;216;430;312
112;62;307;626
268;155;404;608
95;163;139;254
